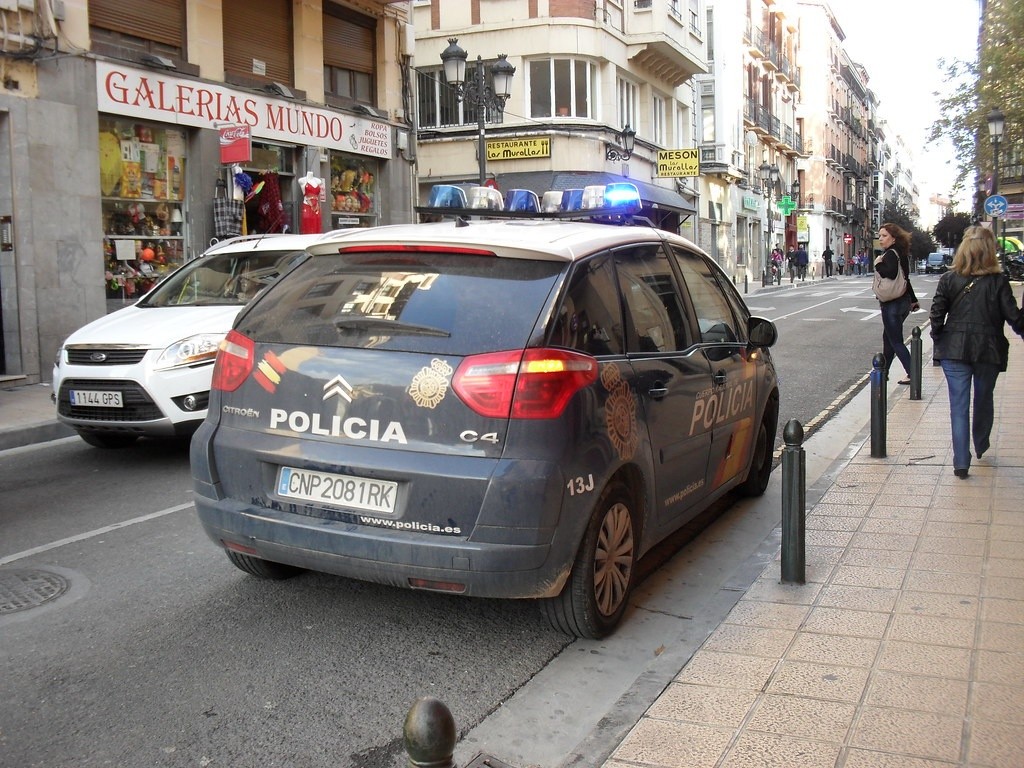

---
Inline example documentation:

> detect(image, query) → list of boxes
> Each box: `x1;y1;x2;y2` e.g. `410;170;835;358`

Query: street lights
759;158;781;288
438;35;515;188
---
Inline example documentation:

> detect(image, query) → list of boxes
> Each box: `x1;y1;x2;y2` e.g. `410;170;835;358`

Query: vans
925;253;946;272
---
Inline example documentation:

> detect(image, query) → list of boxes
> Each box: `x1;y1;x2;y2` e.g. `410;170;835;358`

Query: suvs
48;224;367;455
190;182;781;638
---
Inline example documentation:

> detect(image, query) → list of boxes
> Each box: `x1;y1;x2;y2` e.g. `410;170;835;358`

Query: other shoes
897;380;910;385
954;469;968;479
977;443;990;459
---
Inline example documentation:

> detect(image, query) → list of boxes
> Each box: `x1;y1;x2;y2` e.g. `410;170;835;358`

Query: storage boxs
99;119;186;201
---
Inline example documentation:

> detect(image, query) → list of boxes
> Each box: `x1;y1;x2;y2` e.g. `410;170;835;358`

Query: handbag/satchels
872;249;907;302
836;265;840;271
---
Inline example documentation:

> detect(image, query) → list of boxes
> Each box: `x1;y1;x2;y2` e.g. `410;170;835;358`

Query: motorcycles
997;251;1020;282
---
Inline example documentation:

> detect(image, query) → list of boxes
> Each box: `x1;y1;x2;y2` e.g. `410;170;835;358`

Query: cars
917;261;927;274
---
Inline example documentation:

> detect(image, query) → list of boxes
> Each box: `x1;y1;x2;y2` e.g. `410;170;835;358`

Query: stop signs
844;234;853;245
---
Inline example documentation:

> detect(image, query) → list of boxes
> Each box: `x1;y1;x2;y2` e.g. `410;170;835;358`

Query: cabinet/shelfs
331;188;377;227
100;196;184;278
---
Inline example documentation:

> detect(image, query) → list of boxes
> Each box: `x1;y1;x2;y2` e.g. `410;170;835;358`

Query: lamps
143;54;177;71
354;104;379;118
267;83;294;100
606;124;636;161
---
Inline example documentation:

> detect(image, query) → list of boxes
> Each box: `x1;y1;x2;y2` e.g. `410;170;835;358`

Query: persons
837;253;846;275
872;223;920;385
769;243;809;283
219;259;280;305
850;250;863;275
822;246;834;279
298;171;323;234
929;226;1024;479
330;169;375;215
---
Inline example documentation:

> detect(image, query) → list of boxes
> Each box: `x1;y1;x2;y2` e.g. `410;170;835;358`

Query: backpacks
850;258;855;265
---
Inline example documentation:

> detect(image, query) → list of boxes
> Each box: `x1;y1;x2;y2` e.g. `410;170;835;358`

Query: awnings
494;171;698;228
